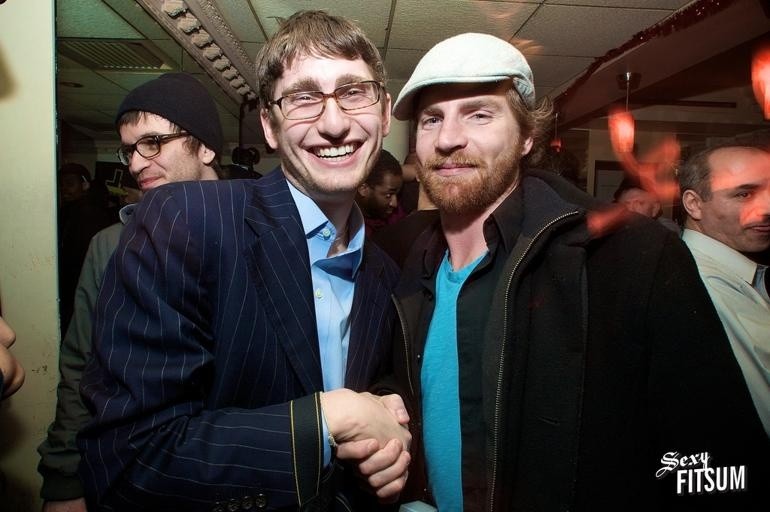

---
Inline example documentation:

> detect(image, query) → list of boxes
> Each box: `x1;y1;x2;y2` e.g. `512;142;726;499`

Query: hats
116;72;225;161
392;32;536;122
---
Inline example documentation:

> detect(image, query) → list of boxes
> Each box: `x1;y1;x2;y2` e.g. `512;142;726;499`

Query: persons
356;148;420;237
86;13;413;509
35;72;225;511
336;33;770;512
1;306;24;423
58;163;105;334
675;144;770;440
610;172;681;236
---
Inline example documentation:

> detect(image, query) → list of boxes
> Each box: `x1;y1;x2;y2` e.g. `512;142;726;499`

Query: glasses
265;80;385;120
116;130;191;167
